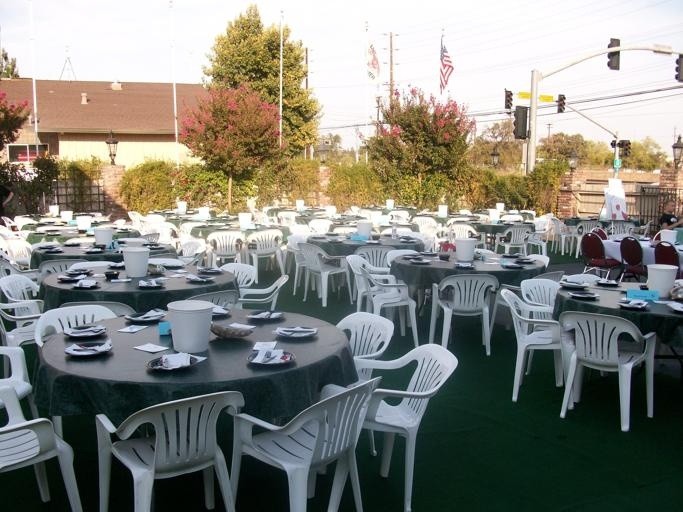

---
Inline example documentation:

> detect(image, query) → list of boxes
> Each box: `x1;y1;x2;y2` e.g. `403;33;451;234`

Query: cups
238;199;505;251
659;229;676;246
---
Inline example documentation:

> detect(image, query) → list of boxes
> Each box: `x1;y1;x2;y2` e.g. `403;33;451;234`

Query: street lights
672;135;683;219
489;144;500;166
564;147;581;176
106;131;121;165
318;139;332;163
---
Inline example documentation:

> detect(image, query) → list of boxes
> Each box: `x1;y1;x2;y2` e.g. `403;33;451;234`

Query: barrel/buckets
49;199;505;278
660;229;679;246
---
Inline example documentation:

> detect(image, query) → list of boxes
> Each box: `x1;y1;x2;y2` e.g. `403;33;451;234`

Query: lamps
105;129;118;165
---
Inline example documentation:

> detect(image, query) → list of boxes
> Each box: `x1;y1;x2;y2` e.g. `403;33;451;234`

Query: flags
364;26;380;80
439;40;454;94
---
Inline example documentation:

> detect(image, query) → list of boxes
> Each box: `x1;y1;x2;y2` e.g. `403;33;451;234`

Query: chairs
1;386;82;512
559;311;657;432
230;376;384;511
1;206;682;348
334;311;395;457
95;391;244;512
501;287;581;410
428;273;499;355
1;345;39;421
319;343;459;512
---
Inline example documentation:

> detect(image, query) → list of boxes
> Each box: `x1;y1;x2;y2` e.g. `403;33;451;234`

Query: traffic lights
624;142;631;155
611;139;631;148
504;91;513;109
675;54;683;82
607;38;620;71
512;106;527;140
558;94;566;113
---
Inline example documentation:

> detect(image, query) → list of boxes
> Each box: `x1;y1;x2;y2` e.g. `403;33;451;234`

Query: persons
658;198;682;230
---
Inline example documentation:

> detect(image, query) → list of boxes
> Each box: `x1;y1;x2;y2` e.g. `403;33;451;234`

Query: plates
556;277;682;316
611;235;683;253
60;308;319;373
400;251;537;271
28;201;237;290
